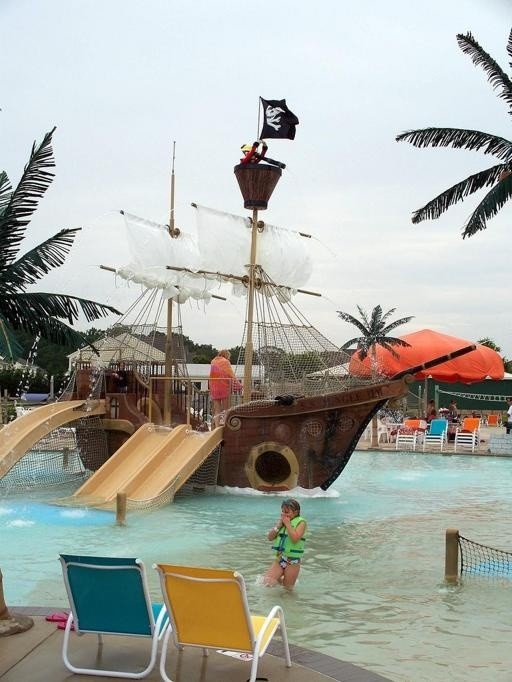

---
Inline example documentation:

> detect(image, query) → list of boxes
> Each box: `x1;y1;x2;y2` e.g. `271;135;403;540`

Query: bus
31;428;77;450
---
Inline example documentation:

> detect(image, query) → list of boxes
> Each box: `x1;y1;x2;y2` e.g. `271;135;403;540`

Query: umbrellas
349;328;505;418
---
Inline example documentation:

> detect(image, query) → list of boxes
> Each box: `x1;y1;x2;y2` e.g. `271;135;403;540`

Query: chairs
423;419;448;453
152;563;292;682
487;415;498;427
375;419;389;443
57;553;170;680
454;417;481;453
395;420;421;451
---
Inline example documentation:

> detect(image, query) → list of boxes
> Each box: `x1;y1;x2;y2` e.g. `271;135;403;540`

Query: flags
259;97;299;141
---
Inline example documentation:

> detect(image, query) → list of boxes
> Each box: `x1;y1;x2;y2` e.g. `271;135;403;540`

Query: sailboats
0;96;477;510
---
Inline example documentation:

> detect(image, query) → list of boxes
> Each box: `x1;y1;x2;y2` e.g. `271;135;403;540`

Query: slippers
56;620;75;629
44;613;70;622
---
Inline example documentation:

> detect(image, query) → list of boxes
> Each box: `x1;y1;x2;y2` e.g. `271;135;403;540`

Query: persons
262;499;308;598
449;400;457;419
425;399;435;419
209;349;244;431
427;409;437;423
503;397;512;434
